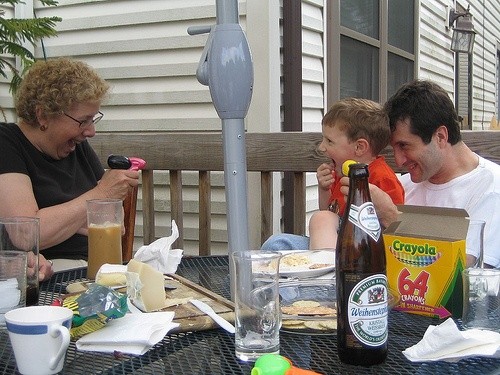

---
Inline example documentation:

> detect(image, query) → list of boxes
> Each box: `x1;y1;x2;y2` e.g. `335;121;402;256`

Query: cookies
277;300;338;330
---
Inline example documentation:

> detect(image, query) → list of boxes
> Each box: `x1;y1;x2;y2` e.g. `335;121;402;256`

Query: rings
48;260;54;265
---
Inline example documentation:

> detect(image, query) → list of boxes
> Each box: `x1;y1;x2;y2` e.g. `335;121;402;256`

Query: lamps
447;5;477;54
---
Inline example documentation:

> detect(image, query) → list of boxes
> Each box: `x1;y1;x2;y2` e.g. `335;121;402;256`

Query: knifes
191;299;237;333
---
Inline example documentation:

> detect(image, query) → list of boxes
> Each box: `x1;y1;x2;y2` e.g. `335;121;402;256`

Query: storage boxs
383;204;477;321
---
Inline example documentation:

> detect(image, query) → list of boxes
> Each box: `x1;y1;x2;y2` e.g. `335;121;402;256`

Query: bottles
335;163;388;364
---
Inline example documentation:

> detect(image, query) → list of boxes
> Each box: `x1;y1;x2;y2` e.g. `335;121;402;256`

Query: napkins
75;310;181;355
94;219;183;289
402;317;500;362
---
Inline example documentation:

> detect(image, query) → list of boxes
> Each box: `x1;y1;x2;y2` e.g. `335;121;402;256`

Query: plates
248;278;338;335
252;249;335;279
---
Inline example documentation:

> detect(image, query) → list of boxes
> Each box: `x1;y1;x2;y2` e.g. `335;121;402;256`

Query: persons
0;251;54;282
261;81;500;370
309;98;405;249
0;58;140;261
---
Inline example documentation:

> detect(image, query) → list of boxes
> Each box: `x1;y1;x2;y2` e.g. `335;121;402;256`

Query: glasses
64;111;104;129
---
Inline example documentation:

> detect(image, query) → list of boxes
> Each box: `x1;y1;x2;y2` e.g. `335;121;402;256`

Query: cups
461;268;500;333
0;249;32;363
0;216;41;305
466;220;488;300
86;198;125;279
231;250;280;362
5;305;74;375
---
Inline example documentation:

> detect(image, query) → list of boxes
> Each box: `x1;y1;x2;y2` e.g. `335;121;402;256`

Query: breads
309;263;334;269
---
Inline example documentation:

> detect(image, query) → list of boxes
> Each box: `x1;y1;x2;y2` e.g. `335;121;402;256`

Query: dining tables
0;254;500;375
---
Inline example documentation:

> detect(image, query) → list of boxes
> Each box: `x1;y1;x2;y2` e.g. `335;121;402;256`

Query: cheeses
125;259;166;313
95;263;128;287
